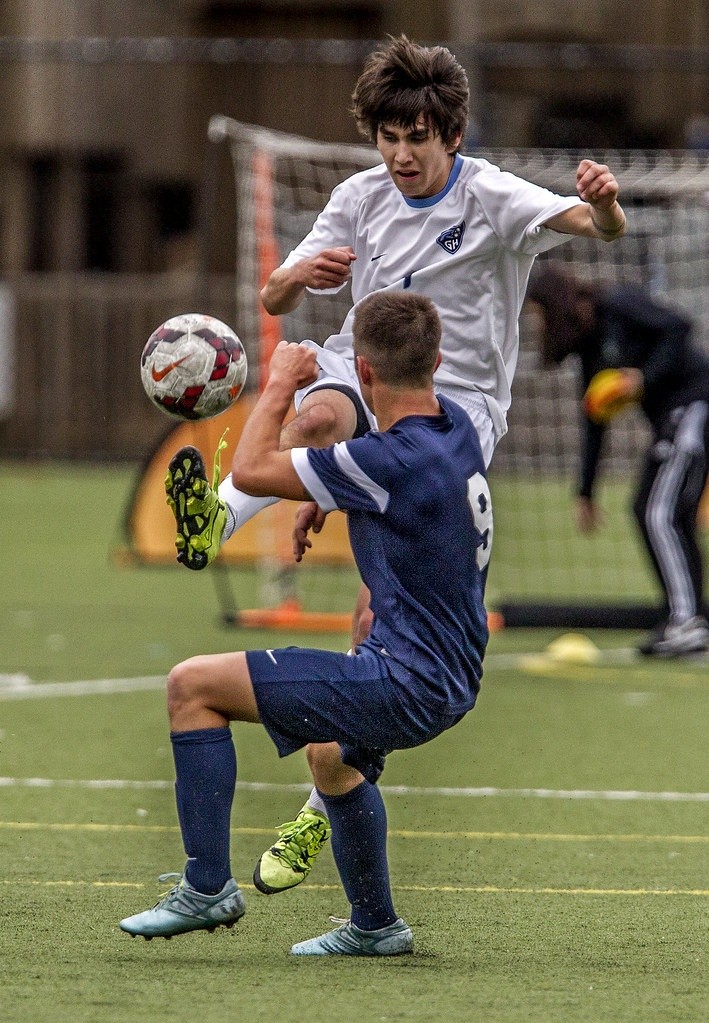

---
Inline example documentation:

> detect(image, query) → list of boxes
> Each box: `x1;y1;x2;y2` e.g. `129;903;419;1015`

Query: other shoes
636;615;708;657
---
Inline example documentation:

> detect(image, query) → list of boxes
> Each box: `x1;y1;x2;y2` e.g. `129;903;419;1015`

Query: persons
165;34;627;893
117;293;496;954
526;265;709;661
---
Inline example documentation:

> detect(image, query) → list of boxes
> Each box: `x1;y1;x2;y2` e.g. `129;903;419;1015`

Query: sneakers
292;916;413;957
253;805;332;895
164;428;231;571
119;858;245;941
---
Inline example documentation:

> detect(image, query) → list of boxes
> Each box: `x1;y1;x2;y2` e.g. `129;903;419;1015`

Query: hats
528;272;574;365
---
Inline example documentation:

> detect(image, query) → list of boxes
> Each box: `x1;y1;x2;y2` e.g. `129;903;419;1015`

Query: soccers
140;313;247;422
